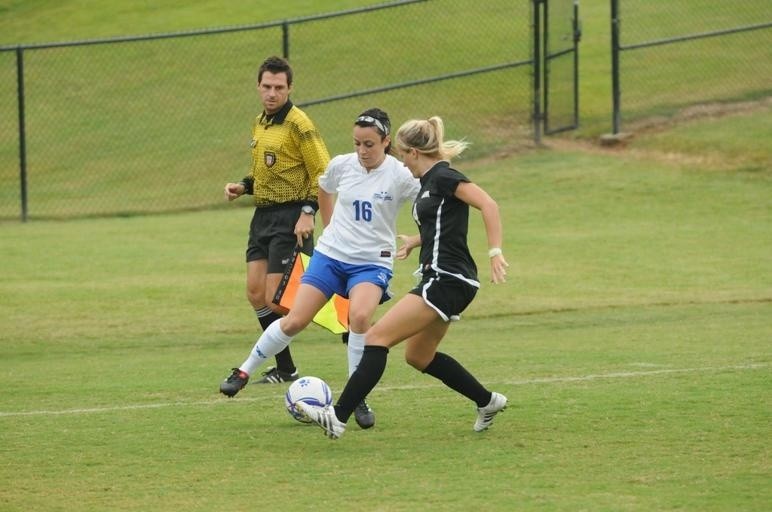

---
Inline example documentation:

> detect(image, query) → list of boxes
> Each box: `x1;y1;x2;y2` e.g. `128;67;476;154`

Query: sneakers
295;397;375;440
251;366;298;385
220;368;249;398
473;392;508;433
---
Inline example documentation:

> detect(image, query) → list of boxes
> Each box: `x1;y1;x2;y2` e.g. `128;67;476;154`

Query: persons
295;116;509;440
224;56;330;383
220;108;421;429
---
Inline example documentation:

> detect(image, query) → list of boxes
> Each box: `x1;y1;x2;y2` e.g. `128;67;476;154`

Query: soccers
285;376;333;423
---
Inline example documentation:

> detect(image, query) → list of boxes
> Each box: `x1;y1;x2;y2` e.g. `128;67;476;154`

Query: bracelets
489;248;502;258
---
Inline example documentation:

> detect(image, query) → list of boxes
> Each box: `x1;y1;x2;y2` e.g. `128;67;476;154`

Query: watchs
302;205;316;216
